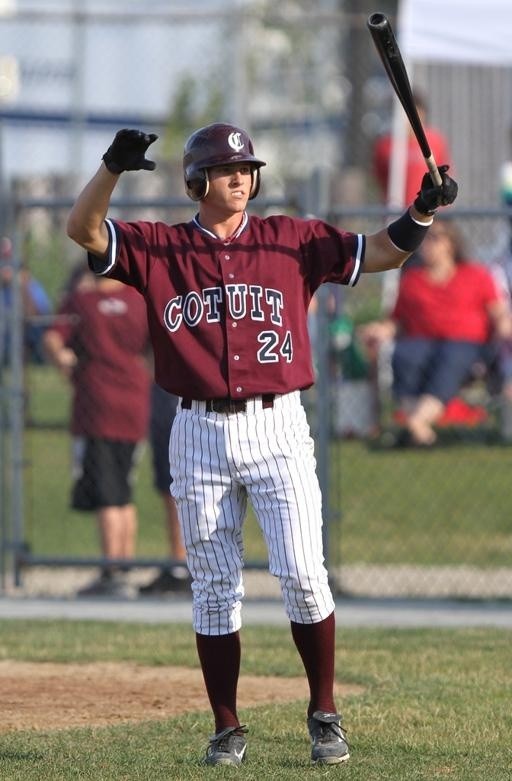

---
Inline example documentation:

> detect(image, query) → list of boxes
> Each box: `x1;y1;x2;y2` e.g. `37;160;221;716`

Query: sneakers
307;711;351;763
142;566;193;598
205;725;250;769
77;568;139;602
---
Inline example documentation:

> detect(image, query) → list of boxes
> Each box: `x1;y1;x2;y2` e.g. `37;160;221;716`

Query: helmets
182;124;265;200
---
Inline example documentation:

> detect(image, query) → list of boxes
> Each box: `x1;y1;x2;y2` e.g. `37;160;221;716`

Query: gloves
101;128;158;175
413;166;458;217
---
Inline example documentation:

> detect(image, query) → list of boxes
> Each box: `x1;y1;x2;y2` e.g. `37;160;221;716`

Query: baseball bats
368;13;451;206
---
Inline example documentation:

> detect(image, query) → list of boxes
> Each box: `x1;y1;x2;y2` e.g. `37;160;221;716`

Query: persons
66;123;457;768
307;84;512;453
0;231;195;604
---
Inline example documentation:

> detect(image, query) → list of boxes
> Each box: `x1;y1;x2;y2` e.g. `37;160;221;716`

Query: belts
181;393;273;411
